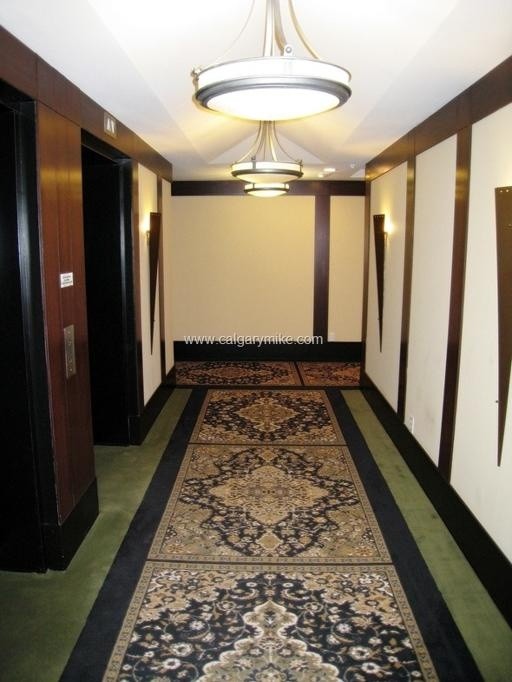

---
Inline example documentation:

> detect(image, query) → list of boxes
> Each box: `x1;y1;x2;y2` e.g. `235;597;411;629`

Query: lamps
242;183;290;198
494;183;512;468
190;1;352;122
372;213;388;354
229;122;304;184
145;208;162;355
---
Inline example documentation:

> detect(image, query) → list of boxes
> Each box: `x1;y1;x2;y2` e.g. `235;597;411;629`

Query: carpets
175;361;361;389
58;389;483;682
